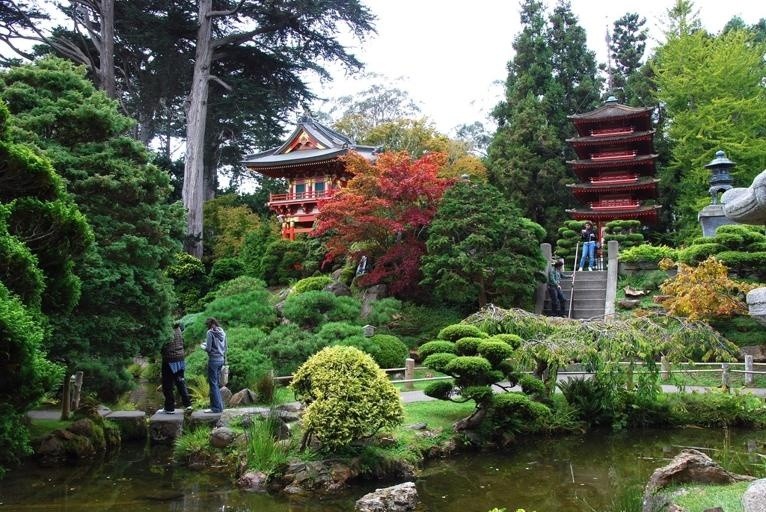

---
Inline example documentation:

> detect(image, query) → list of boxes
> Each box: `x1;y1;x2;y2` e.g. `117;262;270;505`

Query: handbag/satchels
219;365;229;386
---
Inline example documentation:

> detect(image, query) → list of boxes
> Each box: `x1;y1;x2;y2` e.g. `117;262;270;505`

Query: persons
160;325;194;418
547;260;577;317
578;222;597;272
200;317;227;413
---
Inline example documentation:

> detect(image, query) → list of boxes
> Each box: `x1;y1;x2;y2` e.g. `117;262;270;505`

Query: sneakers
157;409;175;415
184;406;194;415
578;266;593;272
203;406;223;413
